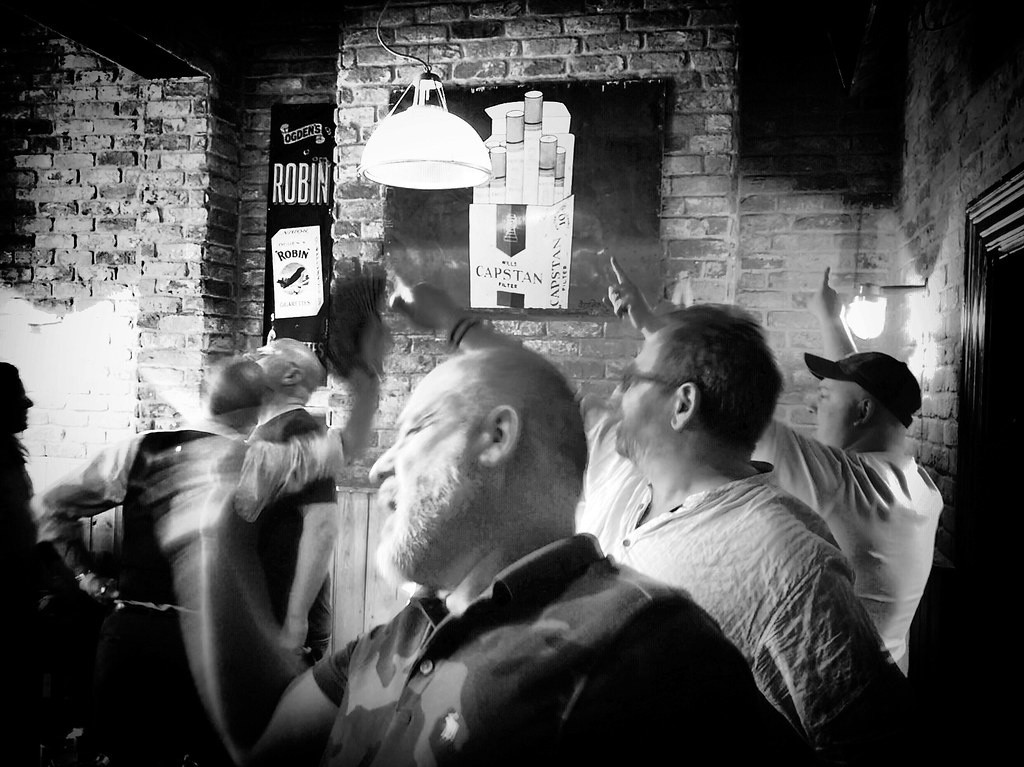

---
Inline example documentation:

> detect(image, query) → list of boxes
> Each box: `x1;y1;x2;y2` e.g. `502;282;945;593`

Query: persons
0;257;1024;767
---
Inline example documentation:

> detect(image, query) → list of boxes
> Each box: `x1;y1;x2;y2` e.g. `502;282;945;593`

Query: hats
803;351;923;430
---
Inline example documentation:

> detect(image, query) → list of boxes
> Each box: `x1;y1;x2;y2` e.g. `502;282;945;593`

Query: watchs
347;358;385;378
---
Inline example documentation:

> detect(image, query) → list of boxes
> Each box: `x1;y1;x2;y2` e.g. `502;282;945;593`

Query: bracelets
454;317;481;349
74;569;92;582
447;314;473;346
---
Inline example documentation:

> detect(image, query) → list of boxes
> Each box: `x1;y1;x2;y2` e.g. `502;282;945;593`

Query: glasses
618;362;679;394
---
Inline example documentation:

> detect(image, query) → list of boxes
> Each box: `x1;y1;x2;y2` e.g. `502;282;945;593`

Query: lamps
358;0;494;189
845;276;935;339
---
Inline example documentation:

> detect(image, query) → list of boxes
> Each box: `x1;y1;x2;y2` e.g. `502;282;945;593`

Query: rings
615;290;620;300
